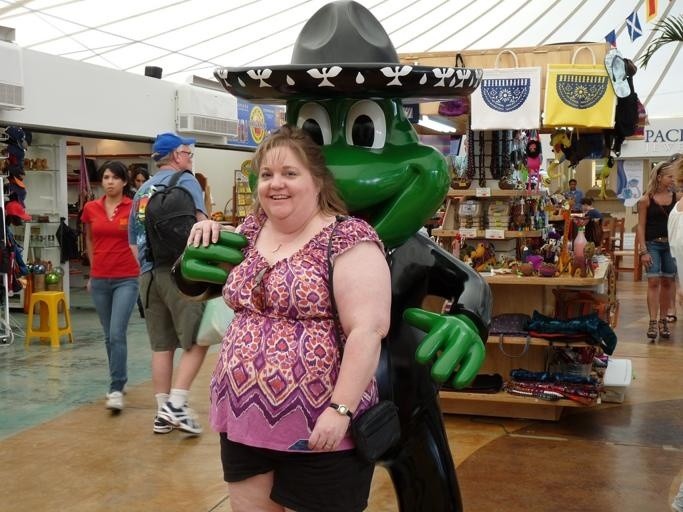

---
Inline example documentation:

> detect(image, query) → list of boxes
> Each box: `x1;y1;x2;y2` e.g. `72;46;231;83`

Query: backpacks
145;169;196;308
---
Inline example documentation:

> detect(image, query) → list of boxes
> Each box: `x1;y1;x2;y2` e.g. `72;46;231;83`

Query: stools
23;290;73;353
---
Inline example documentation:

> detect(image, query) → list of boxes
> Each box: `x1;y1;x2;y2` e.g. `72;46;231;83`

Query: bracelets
638;246;647;255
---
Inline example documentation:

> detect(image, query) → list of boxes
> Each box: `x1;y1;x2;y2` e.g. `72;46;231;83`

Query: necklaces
273;242;284;252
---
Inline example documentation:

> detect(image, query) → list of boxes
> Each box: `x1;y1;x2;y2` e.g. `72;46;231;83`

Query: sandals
647;315;676;338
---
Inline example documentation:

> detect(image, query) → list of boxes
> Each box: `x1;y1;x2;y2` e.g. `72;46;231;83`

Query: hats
5;126;32;221
152;133;196;161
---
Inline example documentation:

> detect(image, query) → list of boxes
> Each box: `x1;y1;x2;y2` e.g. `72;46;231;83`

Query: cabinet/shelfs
8;145;62;248
421;187;611;422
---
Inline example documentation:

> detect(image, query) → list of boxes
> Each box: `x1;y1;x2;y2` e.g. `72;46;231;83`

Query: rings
324;443;334;450
195;227;203;231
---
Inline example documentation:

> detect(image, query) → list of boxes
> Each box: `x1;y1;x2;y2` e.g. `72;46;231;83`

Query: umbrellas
76;145;90;192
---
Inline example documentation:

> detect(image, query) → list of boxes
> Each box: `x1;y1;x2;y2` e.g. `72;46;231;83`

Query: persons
130;165;150;190
667;157;683;291
182;125;399;511
634;159;679;343
127;131;211;438
665;275;679;324
81;159;142;414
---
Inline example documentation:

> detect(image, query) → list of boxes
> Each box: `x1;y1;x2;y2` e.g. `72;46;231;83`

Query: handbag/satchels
439;50;540;130
585;221;603;247
543;46;618;129
490;314;531;357
616;93;649;140
351;400;400;462
0;228;28;292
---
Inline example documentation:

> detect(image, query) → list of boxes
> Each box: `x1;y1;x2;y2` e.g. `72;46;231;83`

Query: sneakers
107;391;123;410
153;401;202;433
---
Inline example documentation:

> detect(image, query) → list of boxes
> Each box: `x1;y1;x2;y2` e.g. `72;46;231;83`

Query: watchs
328;403;353;419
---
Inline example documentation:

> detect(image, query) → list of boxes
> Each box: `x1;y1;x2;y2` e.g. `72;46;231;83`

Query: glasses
181;151;193;158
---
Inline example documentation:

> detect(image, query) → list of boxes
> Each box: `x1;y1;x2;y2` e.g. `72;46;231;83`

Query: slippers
605;48;631;98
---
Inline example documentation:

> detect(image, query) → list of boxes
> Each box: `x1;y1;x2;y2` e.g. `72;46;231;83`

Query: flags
645;0;662;22
624;11;644;42
604;27;620;49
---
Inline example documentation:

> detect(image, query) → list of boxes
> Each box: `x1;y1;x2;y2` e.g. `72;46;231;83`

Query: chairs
608;225;642;282
